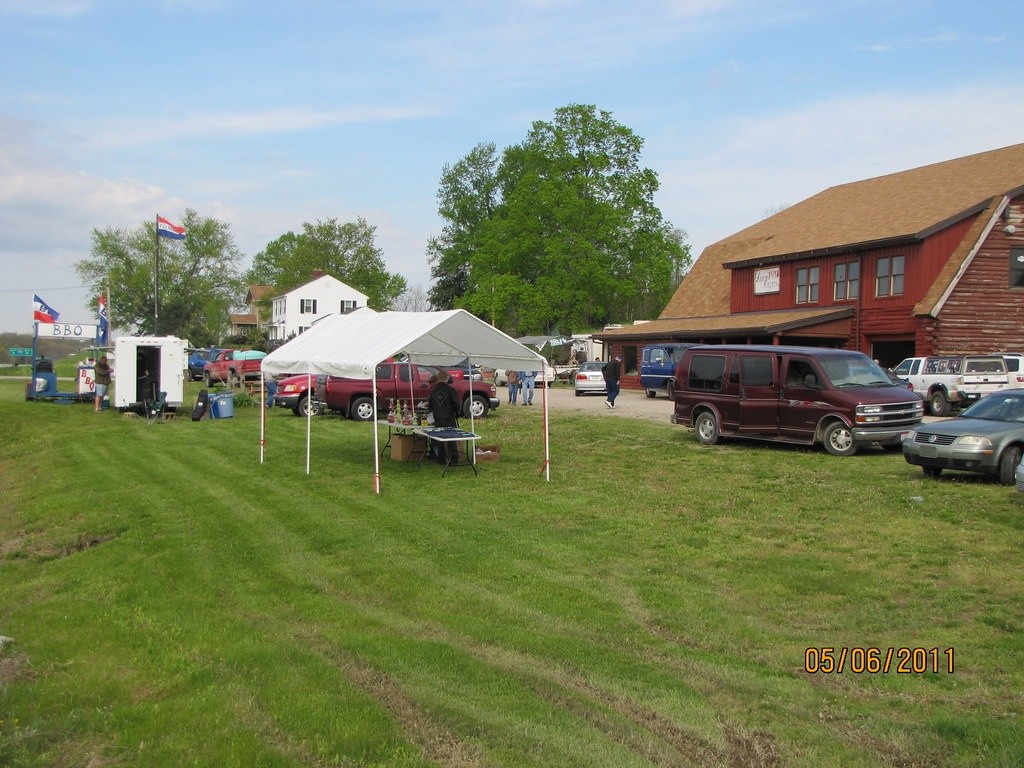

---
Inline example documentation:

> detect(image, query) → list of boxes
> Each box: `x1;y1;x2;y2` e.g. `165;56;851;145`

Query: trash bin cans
208;390;234;418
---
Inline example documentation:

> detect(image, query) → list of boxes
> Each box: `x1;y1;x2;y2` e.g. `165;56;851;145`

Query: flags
157;216;186;239
99;292;108;346
33;293;60;323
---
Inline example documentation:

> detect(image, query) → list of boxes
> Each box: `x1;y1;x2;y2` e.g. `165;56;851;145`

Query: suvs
458;357;483;381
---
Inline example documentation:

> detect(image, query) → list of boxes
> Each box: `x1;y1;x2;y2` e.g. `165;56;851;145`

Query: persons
137;352;148;381
601;355;623;408
505;370;519;405
428;371;462;465
518;370;538;406
575;347;587;364
873;359;879;365
596;357;600;362
264;372;279;410
94;356;112;413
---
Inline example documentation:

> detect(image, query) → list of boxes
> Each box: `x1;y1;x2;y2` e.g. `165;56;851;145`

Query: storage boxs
391;434;427;461
476;445;501;461
444;440;464;464
102;396;109;409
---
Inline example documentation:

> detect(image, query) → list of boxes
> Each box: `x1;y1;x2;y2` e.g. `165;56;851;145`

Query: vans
641;343;703;401
670;344;925;457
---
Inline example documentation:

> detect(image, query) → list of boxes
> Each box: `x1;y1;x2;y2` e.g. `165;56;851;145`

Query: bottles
396;399;402;423
387;398;395;423
413;412;419;426
402;400;410;425
421;412;428;426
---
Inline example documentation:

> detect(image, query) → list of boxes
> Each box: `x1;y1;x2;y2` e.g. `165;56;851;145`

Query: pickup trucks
324;361;498;423
202;349;264;388
274;358;464;417
183;349;223;382
889;353;1024;416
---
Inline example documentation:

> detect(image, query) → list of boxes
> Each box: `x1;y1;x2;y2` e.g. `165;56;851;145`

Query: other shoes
529;403;532;405
438;455;449;464
522;403;527;406
512;402;516;405
508;401;511;403
604;400;615;408
450;455;458;466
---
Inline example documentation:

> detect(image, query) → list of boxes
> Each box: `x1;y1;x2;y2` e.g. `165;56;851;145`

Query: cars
574;361;610;397
493;355;556;388
901;388;1024;485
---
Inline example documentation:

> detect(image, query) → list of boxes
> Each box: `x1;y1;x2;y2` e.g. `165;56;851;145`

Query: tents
261;307;549;494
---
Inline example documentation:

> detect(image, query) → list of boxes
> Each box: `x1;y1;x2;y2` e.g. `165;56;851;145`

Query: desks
377;419;435;464
412;426;482;479
